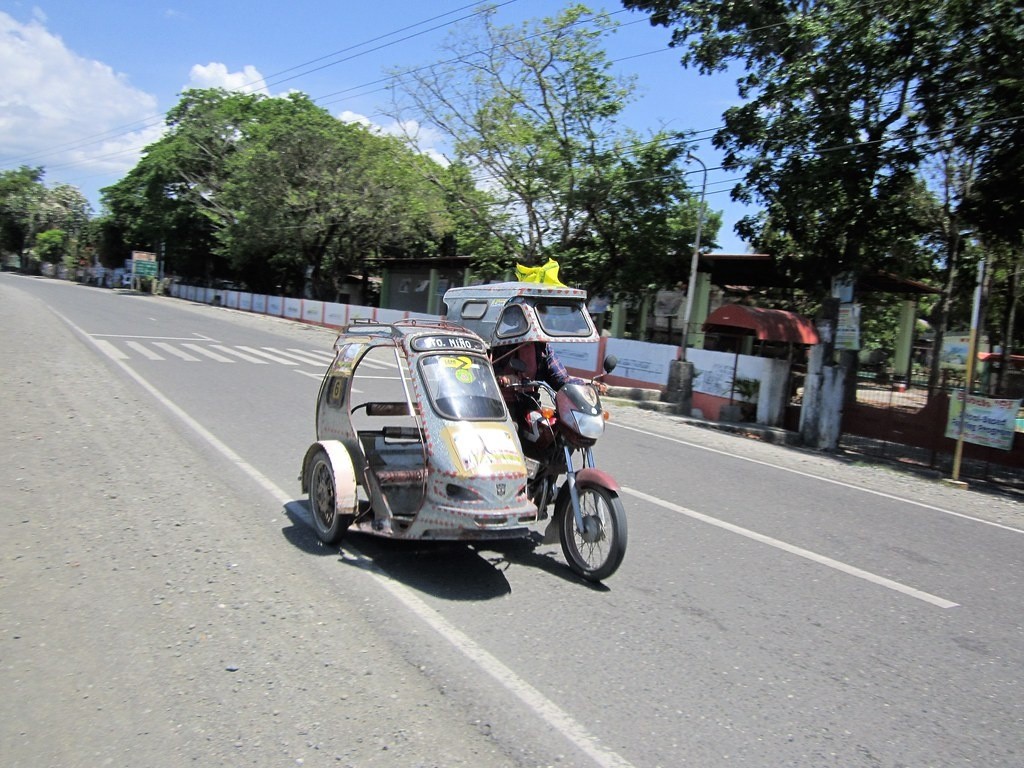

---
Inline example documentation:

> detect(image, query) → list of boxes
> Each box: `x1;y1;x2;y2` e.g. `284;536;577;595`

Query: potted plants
720;376;761;423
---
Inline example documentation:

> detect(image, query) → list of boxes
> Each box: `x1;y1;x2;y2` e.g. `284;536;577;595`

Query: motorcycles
296;260;628;583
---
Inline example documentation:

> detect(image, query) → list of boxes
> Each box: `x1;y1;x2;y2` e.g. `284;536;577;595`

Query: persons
489;303;605;506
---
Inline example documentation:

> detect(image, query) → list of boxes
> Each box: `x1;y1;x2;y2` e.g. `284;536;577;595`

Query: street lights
658;152;707;404
